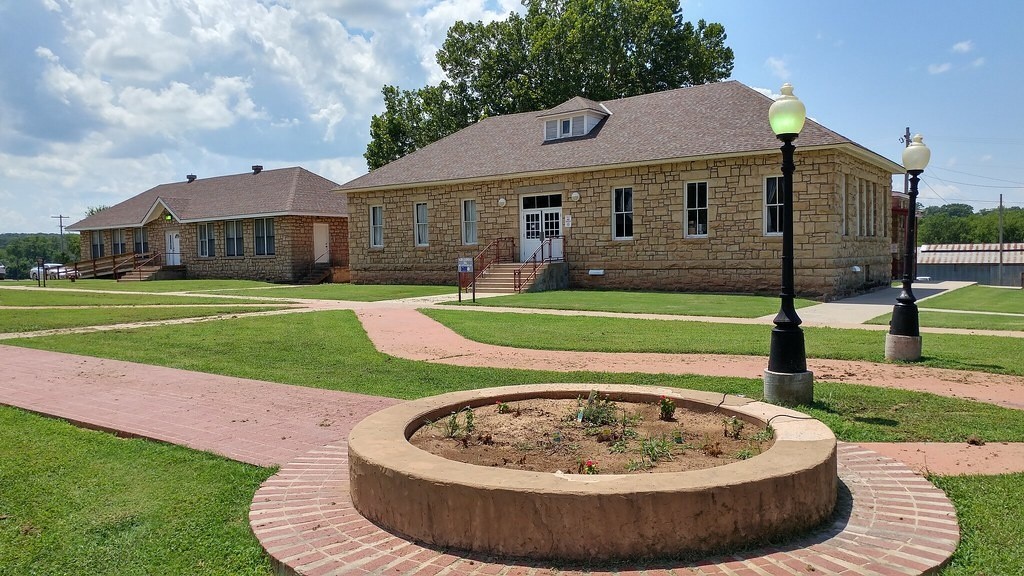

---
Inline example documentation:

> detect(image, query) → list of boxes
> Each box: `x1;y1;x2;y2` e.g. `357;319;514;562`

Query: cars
31;262;78;280
0;262;6;281
29;263;80;279
